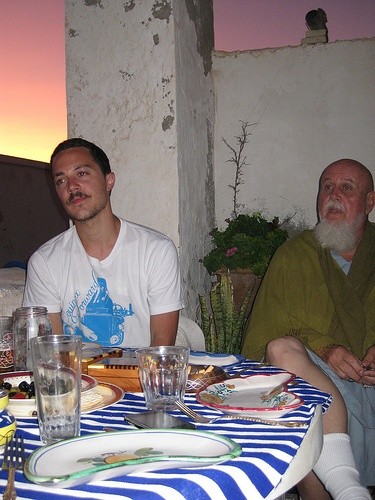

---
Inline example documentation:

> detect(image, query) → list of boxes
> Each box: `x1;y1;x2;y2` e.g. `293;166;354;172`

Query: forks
173;399;306;427
2;434;25;500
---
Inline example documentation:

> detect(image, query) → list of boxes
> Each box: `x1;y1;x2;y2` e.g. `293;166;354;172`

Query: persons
246;159;375;500
23;139;181;349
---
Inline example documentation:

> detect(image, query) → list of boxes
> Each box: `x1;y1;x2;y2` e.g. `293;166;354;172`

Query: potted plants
199;210;299;323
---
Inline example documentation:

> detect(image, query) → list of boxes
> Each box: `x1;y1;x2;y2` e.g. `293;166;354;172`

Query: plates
188;350;246;367
196;371;303;420
24;429;242;488
14;381;125;419
0;369;98;406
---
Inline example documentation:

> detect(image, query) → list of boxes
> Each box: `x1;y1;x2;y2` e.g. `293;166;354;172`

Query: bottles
135;345;190;411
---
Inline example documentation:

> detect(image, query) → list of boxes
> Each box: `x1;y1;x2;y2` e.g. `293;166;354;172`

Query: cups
30;335;82;444
0;316;14;368
12;305;52;371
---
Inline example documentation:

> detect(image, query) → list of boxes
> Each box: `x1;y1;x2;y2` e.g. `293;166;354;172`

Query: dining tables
0;346;333;500
